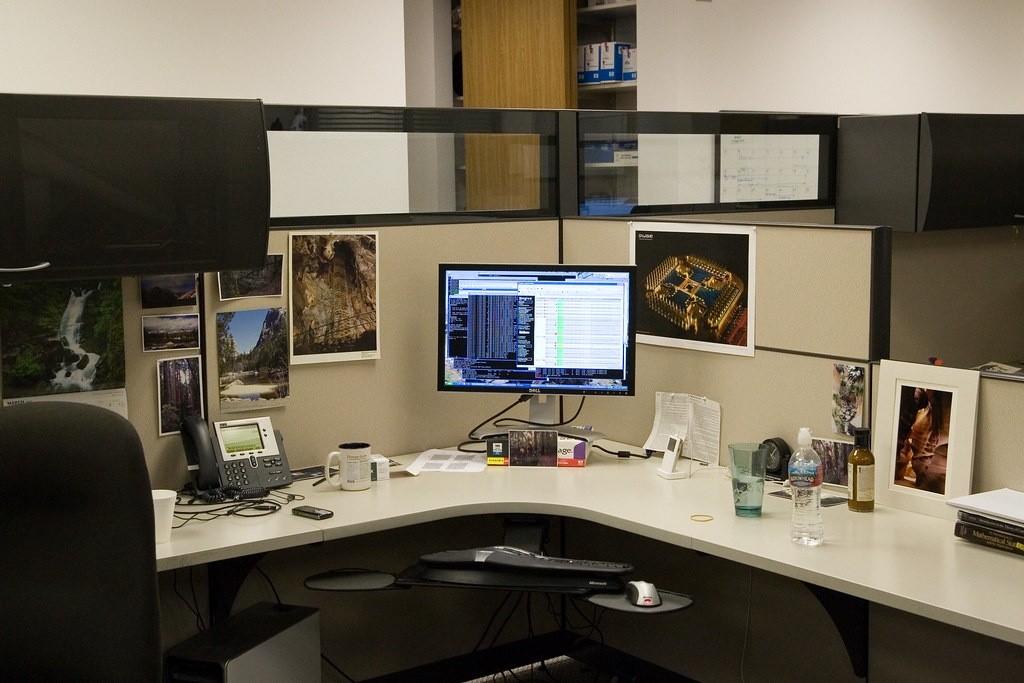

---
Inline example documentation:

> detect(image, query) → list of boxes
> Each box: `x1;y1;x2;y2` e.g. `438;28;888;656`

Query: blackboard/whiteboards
1;0;410;217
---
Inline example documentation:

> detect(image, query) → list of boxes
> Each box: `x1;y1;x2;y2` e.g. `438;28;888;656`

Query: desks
147;431;1024;683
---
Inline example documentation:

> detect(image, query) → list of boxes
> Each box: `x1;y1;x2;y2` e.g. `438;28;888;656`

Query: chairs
0;402;164;683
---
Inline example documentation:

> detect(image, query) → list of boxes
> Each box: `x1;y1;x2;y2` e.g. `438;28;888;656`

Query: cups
324;441;371;491
151;489;176;543
728;442;767;516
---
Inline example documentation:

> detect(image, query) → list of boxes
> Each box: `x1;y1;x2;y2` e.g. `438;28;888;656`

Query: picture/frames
874;358;981;523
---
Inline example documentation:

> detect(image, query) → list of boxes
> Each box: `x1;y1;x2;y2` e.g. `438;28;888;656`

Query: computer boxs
163;601;322;683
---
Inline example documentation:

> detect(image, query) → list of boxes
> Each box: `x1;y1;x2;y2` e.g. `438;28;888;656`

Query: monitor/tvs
437;263;638;441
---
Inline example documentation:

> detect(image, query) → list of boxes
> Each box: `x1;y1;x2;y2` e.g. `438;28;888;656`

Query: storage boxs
577;42;637;86
486;425;594;467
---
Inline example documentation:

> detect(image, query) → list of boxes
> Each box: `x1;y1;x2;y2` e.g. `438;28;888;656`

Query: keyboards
421;545;634;577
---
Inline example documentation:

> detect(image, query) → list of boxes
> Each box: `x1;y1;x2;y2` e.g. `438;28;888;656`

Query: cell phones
292;506;333;520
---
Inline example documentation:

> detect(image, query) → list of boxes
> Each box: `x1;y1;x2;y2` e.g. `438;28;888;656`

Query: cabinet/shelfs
834;112;1024;233
0;93;271;289
451;0;638;212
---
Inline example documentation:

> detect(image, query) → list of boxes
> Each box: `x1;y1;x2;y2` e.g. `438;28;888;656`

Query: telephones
656;430;687;480
181;416;293;498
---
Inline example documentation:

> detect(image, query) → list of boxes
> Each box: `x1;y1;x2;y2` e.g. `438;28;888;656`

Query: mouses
626;580;661;606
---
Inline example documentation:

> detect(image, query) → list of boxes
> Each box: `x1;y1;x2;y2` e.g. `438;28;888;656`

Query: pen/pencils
312;472;336;486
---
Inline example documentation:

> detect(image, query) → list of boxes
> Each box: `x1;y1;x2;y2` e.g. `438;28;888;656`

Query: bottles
788;428;824;546
847;427;875;511
